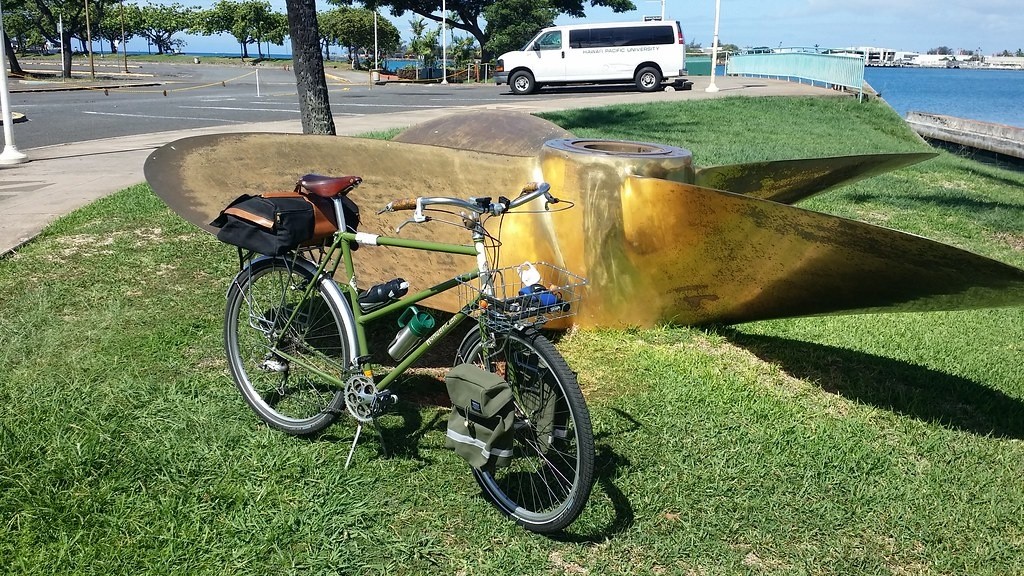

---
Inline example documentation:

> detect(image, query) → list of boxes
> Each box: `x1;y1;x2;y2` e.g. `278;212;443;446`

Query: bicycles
227;174;596;533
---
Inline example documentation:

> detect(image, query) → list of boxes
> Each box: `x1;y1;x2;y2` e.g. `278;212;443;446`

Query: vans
495;20;685;95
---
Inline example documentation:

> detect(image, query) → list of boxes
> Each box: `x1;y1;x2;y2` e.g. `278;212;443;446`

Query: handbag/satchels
443;362;514;471
209;185;360;256
505;351;577;455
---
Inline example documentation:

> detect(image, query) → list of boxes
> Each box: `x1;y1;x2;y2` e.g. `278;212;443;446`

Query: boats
11;40;63;54
171;47;185;56
947;56;960;69
711;51;731;65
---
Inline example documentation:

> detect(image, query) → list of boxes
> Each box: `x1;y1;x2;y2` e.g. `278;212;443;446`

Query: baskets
455;262;588;334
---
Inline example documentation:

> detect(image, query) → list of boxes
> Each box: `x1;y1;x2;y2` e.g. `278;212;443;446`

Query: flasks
386;306;435;361
